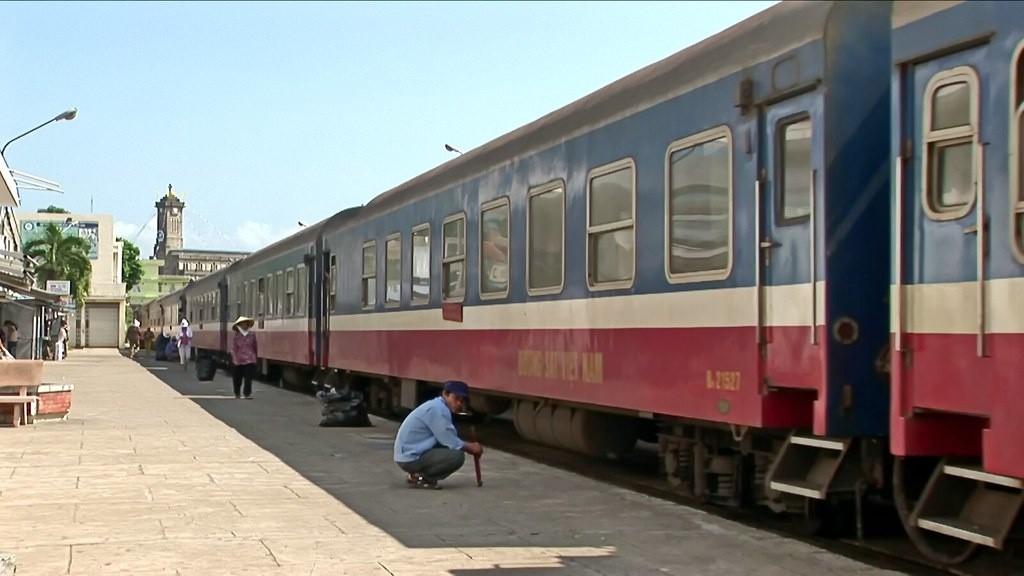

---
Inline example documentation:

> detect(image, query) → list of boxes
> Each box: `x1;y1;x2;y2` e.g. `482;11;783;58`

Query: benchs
0;360;43;427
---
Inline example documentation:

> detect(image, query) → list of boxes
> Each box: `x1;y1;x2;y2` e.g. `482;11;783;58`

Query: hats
131;319;140;327
444;381;470;404
232;316;254;330
180;319;188;327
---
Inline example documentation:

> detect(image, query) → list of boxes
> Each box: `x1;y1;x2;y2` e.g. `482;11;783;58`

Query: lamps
2;107;78;154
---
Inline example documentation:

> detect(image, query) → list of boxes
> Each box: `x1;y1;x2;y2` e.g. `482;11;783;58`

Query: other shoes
235;394;240;399
245;395;252;399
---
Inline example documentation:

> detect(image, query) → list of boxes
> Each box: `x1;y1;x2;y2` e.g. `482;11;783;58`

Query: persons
44;316;70;360
231;316;258;400
394;380;483;489
0;320;19;360
126;318;194;370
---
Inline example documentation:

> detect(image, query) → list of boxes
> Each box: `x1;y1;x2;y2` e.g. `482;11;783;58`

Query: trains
132;0;1024;568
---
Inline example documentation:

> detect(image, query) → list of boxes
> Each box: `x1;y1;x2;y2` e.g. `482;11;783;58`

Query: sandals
407;472;442;491
